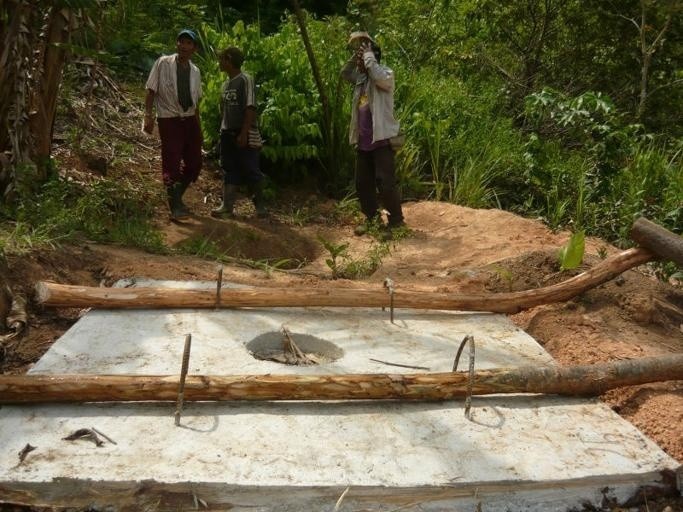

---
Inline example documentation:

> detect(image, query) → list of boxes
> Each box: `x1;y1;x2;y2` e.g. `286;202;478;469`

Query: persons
339;42;405;240
210;47;268;220
144;29;203;220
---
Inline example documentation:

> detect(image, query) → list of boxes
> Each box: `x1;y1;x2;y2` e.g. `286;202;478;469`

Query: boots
211;183;240;216
167;182;191;220
248;182;268;219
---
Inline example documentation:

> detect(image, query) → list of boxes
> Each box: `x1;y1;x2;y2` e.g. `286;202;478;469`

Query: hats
347;32;377;50
177;29;197;42
214;46;244;66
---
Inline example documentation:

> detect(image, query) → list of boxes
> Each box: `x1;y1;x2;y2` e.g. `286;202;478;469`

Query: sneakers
377;215;406;240
354;211;383;235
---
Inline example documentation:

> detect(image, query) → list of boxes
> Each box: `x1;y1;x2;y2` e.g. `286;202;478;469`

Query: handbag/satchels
247;127;263;151
